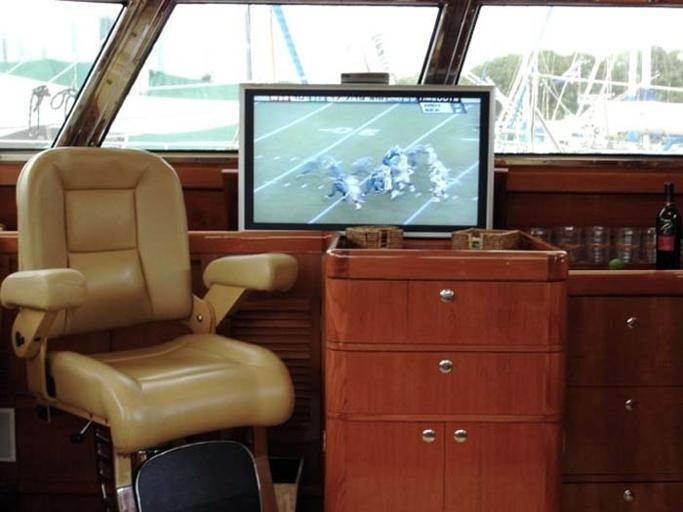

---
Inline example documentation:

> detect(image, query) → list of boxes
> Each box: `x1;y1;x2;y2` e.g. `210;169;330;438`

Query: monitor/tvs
236;81;496;238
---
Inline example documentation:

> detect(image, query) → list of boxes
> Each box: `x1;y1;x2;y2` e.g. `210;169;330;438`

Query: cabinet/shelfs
324;249;568;512
560;271;681;511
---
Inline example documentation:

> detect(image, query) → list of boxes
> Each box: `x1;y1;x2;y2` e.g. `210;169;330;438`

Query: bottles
655;181;682;270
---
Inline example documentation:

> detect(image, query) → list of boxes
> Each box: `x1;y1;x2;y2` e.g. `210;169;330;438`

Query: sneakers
324;184;449;211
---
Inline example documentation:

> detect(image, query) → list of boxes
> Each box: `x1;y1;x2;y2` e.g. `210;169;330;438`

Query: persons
295;143;449;212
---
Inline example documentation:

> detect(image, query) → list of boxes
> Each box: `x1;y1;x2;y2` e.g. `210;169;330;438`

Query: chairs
2;147;298;511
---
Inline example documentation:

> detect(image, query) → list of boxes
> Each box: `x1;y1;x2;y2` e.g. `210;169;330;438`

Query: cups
525;224;656;263
